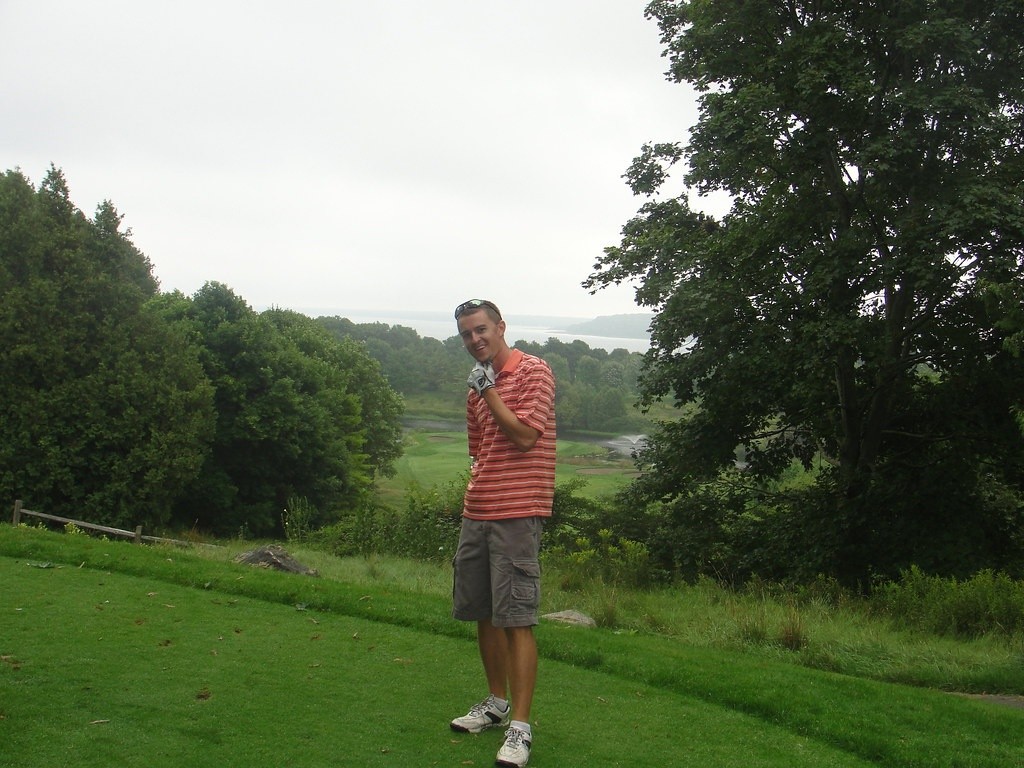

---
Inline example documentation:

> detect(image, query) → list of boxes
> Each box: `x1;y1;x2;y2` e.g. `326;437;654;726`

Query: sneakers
495;726;532;768
450;693;511;734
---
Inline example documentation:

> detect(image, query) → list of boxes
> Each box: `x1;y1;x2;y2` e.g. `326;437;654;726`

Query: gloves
467;361;496;397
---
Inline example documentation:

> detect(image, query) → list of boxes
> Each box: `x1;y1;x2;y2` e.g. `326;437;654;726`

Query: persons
449;299;556;768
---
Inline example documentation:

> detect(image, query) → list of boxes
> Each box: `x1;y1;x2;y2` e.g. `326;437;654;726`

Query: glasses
455;299;502;321
